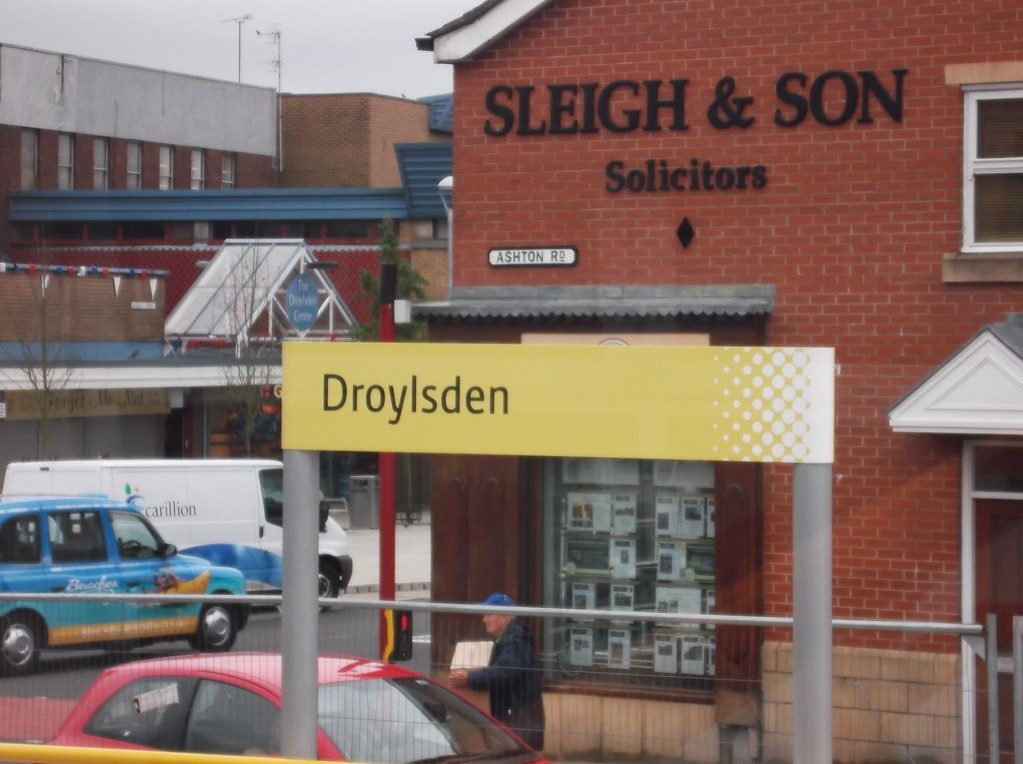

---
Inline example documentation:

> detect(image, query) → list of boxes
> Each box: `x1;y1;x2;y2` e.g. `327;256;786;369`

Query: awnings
408;282;775;319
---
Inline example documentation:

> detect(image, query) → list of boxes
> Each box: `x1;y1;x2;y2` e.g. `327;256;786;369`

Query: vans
0;456;355;616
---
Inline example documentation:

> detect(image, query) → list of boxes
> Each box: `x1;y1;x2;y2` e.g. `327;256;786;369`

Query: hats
482;594;513;606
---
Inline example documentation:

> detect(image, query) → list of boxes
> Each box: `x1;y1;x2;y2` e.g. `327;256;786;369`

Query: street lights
438;176;453;300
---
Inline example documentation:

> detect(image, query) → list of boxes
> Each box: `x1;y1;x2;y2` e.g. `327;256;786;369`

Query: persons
448;596;545;752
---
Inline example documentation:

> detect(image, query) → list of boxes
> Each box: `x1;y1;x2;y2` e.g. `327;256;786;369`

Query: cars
0;648;552;763
0;492;254;674
